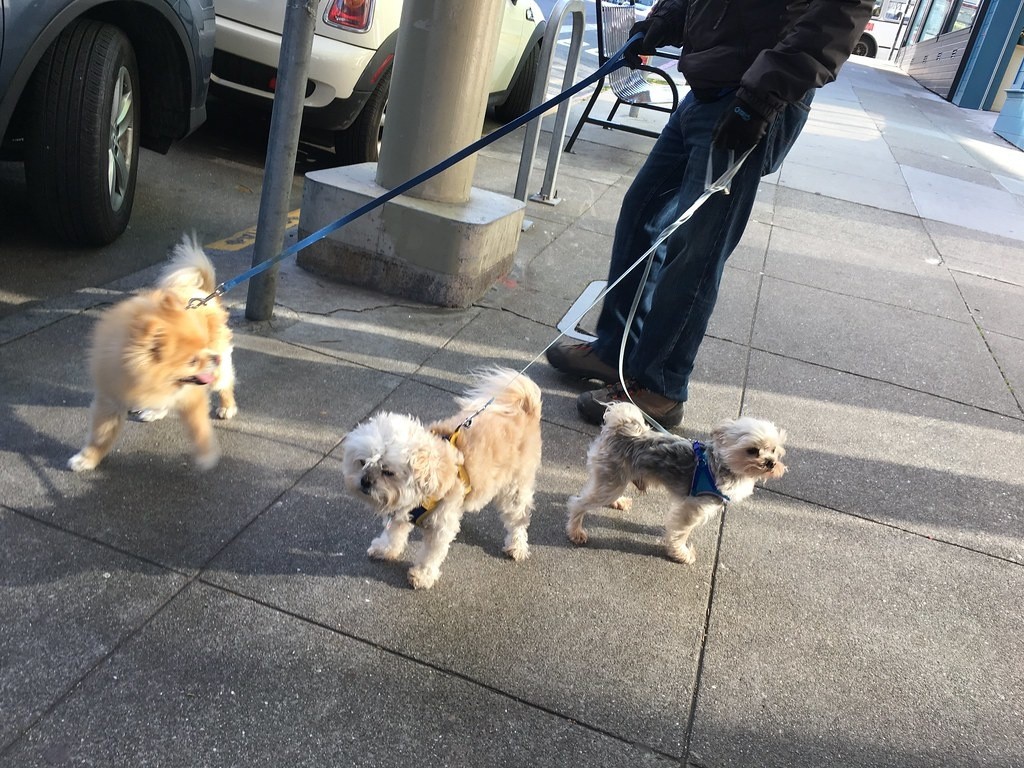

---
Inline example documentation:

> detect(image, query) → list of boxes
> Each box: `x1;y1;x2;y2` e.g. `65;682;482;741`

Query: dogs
68;228;238;473
342;361;543;591
565;399;788;565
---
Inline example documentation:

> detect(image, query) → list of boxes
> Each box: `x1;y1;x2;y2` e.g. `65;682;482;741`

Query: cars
0;0;215;250
208;1;548;167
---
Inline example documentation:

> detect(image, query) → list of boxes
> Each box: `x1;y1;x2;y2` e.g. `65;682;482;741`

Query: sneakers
577;379;684;428
545;342;629;384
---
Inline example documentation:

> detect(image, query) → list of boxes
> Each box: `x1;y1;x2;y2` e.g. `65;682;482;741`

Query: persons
546;0;878;425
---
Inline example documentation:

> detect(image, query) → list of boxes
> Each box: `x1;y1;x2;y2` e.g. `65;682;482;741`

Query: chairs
564;0;681;153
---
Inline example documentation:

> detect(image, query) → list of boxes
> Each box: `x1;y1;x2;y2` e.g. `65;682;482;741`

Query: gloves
711;97;767;150
623;16;669;70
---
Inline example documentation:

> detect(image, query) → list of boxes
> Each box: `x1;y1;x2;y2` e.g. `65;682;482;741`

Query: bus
852;0;981;60
